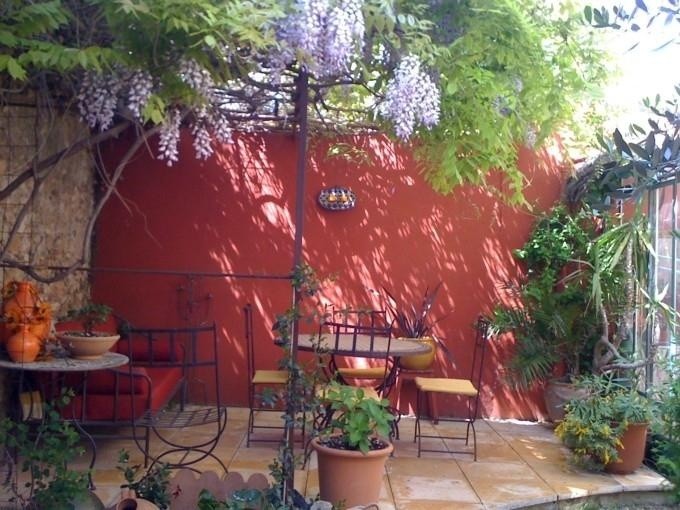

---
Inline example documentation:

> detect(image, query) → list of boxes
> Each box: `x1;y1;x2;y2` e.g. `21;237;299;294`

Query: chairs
316;319;396;435
409;314;490;462
331;305;388;395
241;302;292;450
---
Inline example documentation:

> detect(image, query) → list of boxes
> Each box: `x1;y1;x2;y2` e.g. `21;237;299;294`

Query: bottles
0;279;51;363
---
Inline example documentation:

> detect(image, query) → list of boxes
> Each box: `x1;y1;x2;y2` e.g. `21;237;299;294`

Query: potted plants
385;283;440;367
52;269;121;355
301;381;394;503
485;192;654;476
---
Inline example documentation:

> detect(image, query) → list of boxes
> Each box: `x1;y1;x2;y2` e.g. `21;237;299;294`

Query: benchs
44;320;191;466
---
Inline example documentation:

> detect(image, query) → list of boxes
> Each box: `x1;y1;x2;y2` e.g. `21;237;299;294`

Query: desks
0;353;132;490
270;332;432;455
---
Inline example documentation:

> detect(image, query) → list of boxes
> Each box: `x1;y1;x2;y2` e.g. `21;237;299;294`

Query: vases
4;279;49;362
